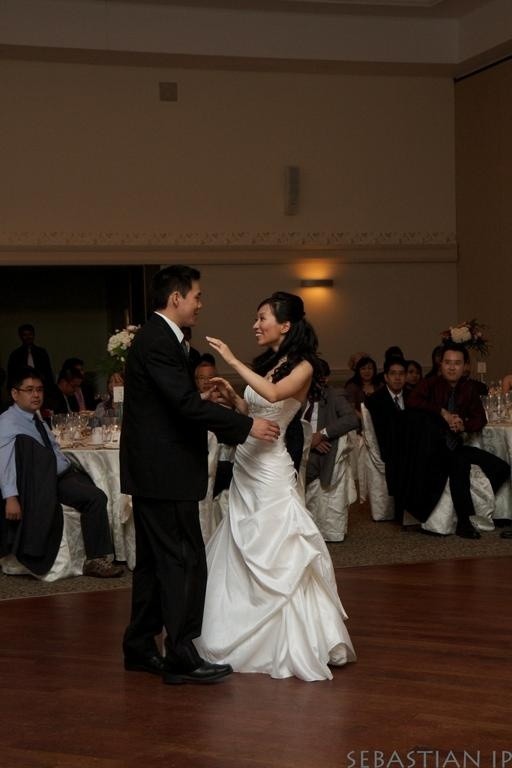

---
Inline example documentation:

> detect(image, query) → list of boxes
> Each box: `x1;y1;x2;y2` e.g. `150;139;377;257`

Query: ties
32;412;52;450
394;396;400;409
180;338;189;359
304;397;314;423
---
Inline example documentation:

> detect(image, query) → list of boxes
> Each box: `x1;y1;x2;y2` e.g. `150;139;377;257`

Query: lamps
299;277;338;291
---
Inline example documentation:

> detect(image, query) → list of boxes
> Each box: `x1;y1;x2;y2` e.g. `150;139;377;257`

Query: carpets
0;498;512;601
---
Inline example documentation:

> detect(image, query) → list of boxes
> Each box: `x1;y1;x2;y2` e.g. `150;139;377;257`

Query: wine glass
51;401;120;448
484;376;512;423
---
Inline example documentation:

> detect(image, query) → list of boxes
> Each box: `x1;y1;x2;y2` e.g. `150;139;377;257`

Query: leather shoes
124;653;165;675
164;662;234;683
500;531;512;539
455;524;480;539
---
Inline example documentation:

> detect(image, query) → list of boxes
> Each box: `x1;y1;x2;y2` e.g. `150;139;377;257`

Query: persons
1;322;512;581
193;290;358;682
118;263;280;685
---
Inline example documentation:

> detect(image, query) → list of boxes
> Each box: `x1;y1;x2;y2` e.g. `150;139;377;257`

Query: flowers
439;317;492;355
109;323;137;367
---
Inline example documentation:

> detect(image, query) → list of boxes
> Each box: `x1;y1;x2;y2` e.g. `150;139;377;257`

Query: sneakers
82;559;125;577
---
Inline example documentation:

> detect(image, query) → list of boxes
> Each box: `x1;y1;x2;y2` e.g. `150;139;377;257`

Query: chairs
121;428;217;570
418;452;500;537
307;422;351;548
214;418;313;536
347;421;365;482
360;398;405;521
0;486;91;585
0;431;110;581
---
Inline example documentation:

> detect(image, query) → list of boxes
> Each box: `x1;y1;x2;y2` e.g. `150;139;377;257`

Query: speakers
285;165;300;217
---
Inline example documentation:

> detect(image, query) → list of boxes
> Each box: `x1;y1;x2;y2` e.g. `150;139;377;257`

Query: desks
43;431;212;569
460;417;512;524
